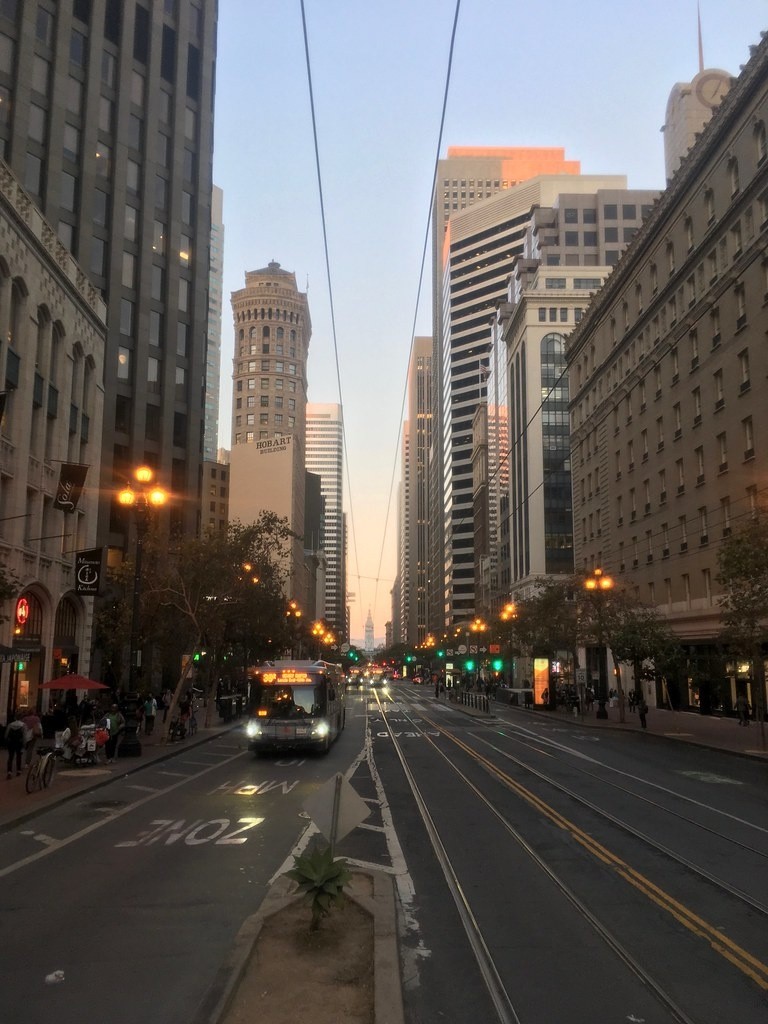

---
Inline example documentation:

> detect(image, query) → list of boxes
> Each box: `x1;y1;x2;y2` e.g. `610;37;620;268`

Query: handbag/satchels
32;716;43;737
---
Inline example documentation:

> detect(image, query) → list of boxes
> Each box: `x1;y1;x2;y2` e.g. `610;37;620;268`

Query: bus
248;658;346;761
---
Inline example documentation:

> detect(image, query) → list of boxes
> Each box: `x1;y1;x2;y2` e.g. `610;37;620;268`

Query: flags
73;547;102;596
53;463;91;515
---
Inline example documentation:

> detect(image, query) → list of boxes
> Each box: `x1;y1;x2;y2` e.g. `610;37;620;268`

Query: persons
476;677;489;696
524;692;533;708
585;685;596;712
96;703;127;765
734;696;753;728
541;687;549;704
623;689;636;713
695;693;699;705
609;688;618;708
5;709;45;780
144;688;192;736
638;698;648;729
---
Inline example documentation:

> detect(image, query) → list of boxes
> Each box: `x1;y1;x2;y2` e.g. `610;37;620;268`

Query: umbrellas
37;671;109;689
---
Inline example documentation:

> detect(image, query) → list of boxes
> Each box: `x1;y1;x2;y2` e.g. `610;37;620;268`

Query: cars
346;664;402;688
412;675;425;685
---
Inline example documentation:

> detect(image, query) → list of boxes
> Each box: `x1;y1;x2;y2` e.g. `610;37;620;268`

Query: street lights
117;465;168;759
586;570;615;719
471;620;486;693
286;602;301;660
499;603;516;688
236;564;259;695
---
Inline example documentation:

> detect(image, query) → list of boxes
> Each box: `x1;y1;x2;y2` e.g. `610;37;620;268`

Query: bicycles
25;747;55;794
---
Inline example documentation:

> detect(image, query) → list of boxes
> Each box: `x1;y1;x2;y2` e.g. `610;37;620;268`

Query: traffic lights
347;651;359;662
437;649;444;657
464;658;475;671
491;656;504;671
406;656;411;663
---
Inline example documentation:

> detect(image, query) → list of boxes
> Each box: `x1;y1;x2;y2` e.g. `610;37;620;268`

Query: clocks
701;80;730;105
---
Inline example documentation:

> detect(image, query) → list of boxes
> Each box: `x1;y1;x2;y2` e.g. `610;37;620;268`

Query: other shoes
6;773;12;779
25;764;30;768
16;772;22;776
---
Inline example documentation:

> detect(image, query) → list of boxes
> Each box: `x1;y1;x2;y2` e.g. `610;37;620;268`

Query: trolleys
73;723;119;769
